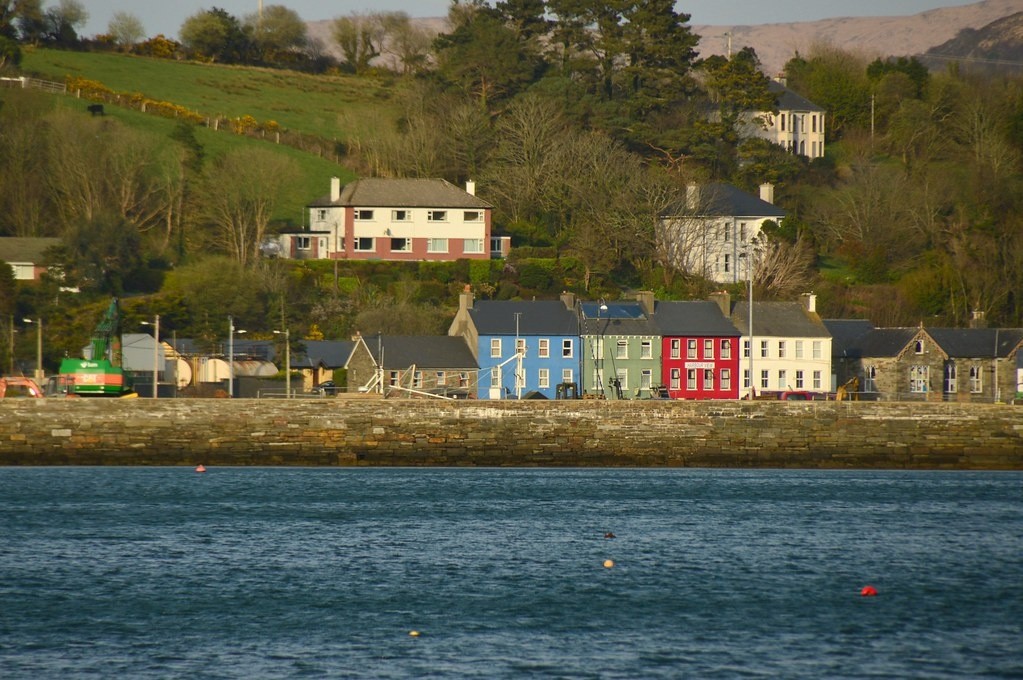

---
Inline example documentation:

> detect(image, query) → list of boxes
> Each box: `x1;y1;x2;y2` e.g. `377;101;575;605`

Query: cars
781;390;811;401
310;380;338;396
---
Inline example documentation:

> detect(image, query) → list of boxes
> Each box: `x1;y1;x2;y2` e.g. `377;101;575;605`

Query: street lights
140;315;160;399
23;318;42;391
273;328;291;398
595;296;608;400
229;318;247;398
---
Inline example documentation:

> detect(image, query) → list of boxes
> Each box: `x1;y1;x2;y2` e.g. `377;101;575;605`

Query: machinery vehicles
835;376;860;401
55;296;125;396
